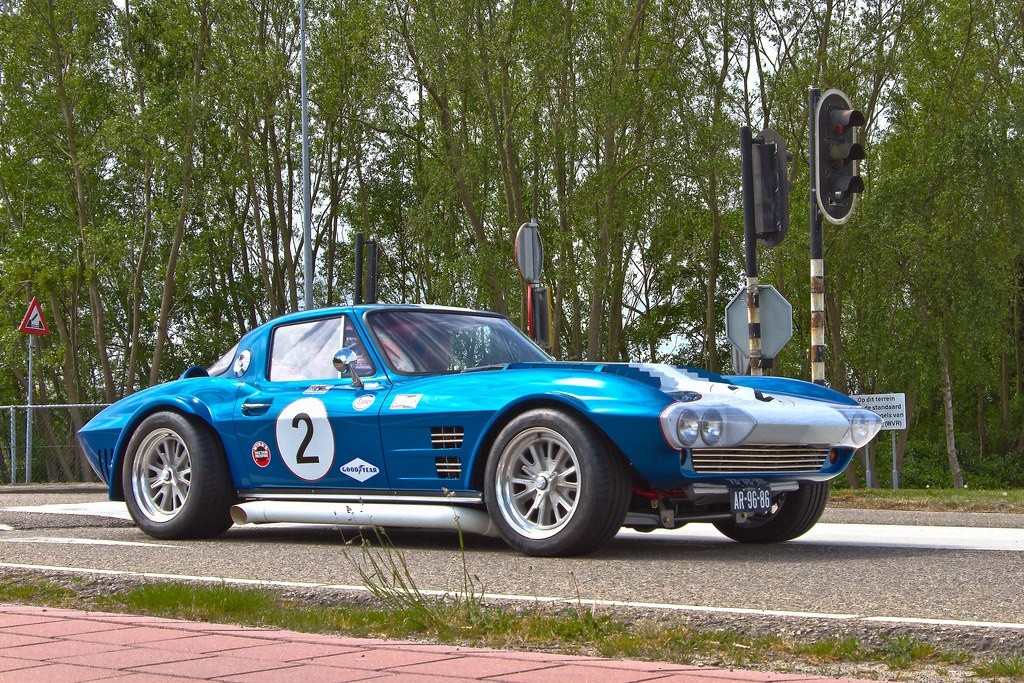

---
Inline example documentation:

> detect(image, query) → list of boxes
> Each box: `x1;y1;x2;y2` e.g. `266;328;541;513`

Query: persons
414;324;454;371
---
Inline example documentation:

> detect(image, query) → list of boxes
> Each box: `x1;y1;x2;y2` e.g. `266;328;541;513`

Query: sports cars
79;301;884;559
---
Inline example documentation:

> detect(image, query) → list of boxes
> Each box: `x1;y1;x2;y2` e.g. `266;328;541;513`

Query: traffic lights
760;128;796;247
813;88;865;224
